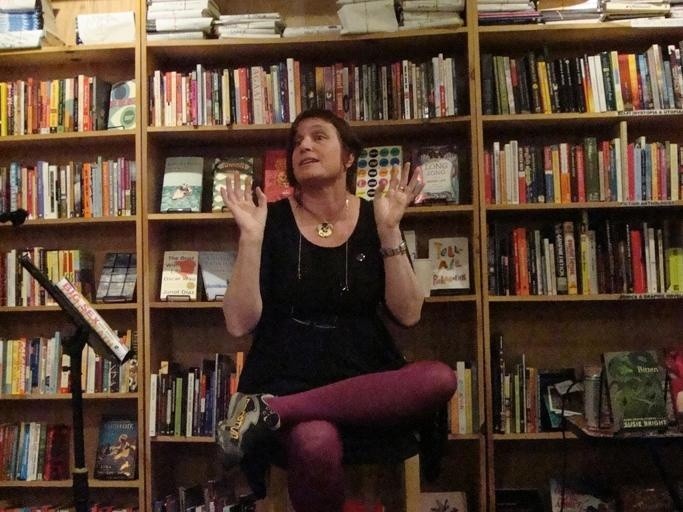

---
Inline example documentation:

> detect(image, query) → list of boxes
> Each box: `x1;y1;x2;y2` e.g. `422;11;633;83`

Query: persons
216;107;457;510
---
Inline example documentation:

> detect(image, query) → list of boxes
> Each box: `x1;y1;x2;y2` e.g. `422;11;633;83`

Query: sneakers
216;391;280;470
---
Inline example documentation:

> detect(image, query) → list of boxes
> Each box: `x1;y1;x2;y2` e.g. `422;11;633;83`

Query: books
157;248;239;306
1;1;137;51
2;418;139;483
404;231;472;293
488;208;682;295
1;77;141;136
446;359;481;435
159;144;457;213
146;1;466;41
481;40;682;117
1;155;138;220
548;443;683;512
2;247;138;396
150;348;247;440
483;120;683;206
477;0;683;29
489;329;683;438
152;480;252;511
151;56;458;125
